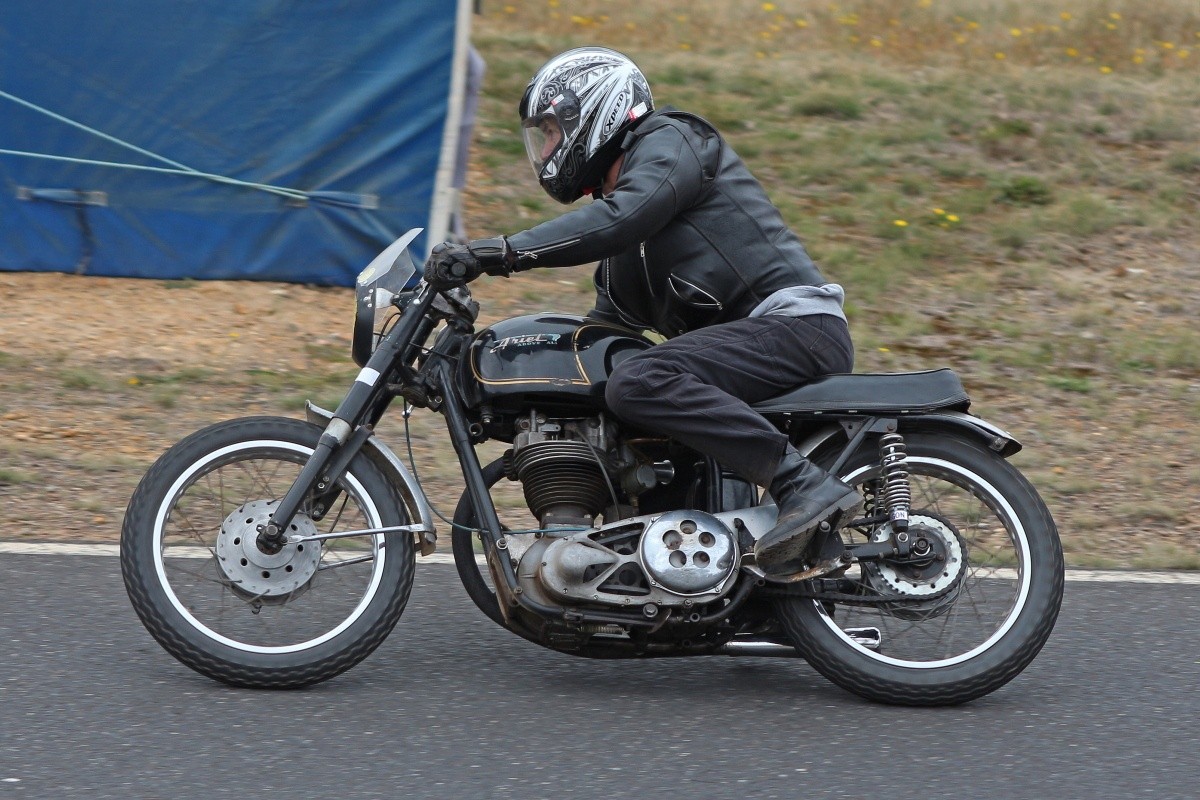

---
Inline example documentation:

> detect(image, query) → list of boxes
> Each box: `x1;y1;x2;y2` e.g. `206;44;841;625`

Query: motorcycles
118;225;1069;708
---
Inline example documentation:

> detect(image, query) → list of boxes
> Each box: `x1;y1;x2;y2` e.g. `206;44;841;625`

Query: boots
752;448;865;573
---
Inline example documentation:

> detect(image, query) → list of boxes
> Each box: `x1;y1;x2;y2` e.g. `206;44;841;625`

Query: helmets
520;46;656;206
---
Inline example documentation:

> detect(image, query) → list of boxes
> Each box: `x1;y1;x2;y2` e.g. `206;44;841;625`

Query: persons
424;46;865;574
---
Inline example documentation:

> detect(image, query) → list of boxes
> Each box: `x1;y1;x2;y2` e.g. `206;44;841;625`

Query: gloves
422;234;511;292
